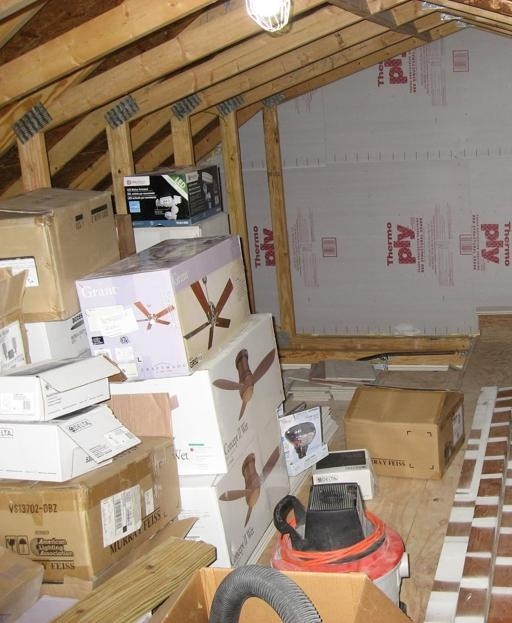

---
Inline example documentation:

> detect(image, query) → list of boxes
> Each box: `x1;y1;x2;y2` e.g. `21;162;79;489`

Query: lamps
245;0;290;33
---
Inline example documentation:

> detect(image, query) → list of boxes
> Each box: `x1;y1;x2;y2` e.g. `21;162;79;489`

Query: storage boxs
344;384;465;479
3;353;124;423
174;410;290;568
117;212;137;256
2;391;185;580
0;437;181;589
124;165;226;228
2;267;32;365
115;313;288;475
73;235;252;380
1;187;119;324
1;406;148;480
133;210;228;254
149;565;413;623
2;548;46;623
